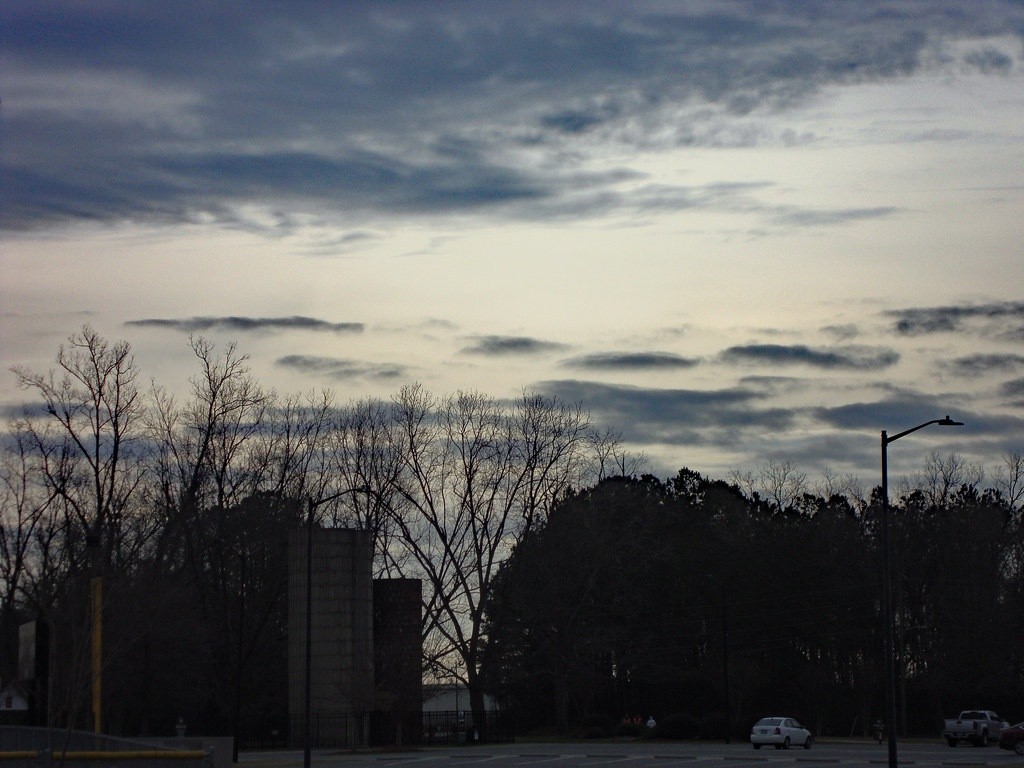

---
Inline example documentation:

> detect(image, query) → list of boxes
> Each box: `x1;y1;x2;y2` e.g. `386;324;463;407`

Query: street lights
302;487;380;768
879;415;965;768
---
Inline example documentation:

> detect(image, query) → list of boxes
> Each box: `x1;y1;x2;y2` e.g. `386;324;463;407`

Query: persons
872;719;885;743
623;712;657;735
999;717;1010;728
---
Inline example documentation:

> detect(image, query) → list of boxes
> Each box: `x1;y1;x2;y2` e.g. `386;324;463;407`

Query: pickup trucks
943;710;1010;749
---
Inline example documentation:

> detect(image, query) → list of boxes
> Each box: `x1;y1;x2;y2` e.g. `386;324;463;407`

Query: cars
997;721;1024;755
751;717;812;750
